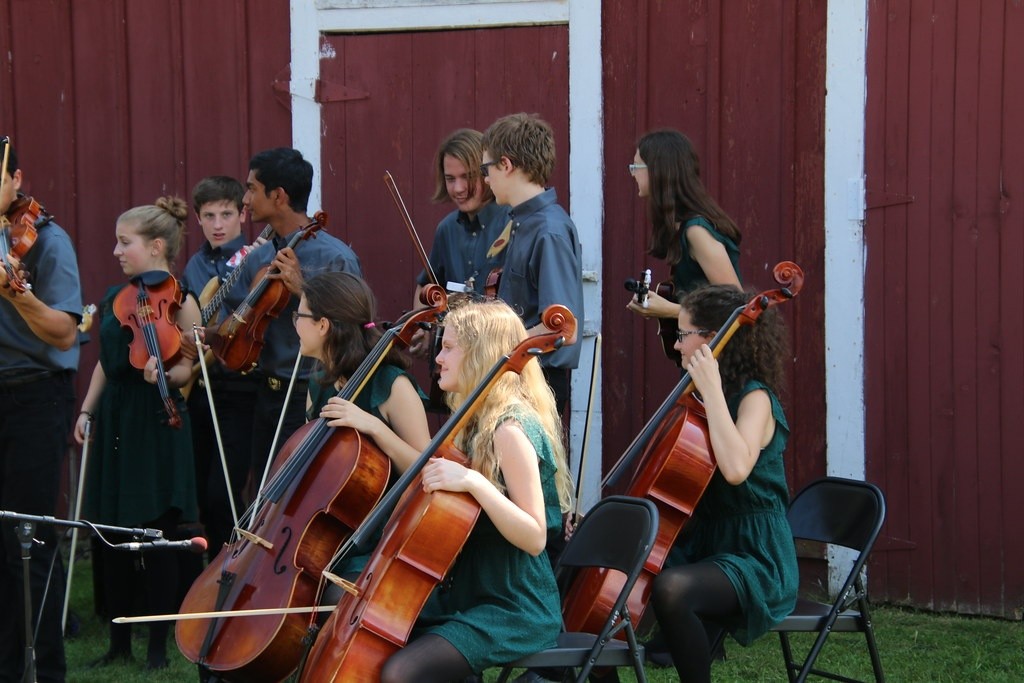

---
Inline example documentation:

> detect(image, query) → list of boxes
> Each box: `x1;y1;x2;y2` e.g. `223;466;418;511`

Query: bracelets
80;410;95;420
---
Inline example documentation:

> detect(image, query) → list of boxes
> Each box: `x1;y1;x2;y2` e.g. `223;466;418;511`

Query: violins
111;269;184;430
484;267;503;298
0;194;42;299
210;209;329;375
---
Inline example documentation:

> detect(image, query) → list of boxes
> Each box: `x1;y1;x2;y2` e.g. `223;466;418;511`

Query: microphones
116;537;208;553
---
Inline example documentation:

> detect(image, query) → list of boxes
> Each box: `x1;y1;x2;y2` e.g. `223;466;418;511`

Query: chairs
494;495;659;683
708;477;887;683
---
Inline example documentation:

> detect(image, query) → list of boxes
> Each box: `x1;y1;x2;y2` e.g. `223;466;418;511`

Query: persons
51;542;78;635
379;298;574;683
74;196;204;670
481;113;585;415
184;148;363;492
624;129;745;668
410;127;513;413
293;271;431;629
0;137;83;683
182;175;268;563
653;283;798;683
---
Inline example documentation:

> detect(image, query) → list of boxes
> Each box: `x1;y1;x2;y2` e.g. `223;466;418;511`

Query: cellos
300;304;576;681
546;259;807;682
174;283;448;681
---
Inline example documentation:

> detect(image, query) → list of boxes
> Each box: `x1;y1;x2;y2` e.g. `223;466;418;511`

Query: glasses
629;163;650;174
291;311;314;326
480;160;500;176
676;329;708;343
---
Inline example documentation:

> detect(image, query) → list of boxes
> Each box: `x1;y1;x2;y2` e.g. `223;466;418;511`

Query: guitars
624;267;682;369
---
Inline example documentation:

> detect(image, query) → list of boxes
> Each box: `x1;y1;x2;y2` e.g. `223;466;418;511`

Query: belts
265;376;309;393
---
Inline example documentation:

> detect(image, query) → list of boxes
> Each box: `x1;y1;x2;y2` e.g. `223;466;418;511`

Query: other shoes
145;652;168;669
91;651;136;667
64;611;81;636
